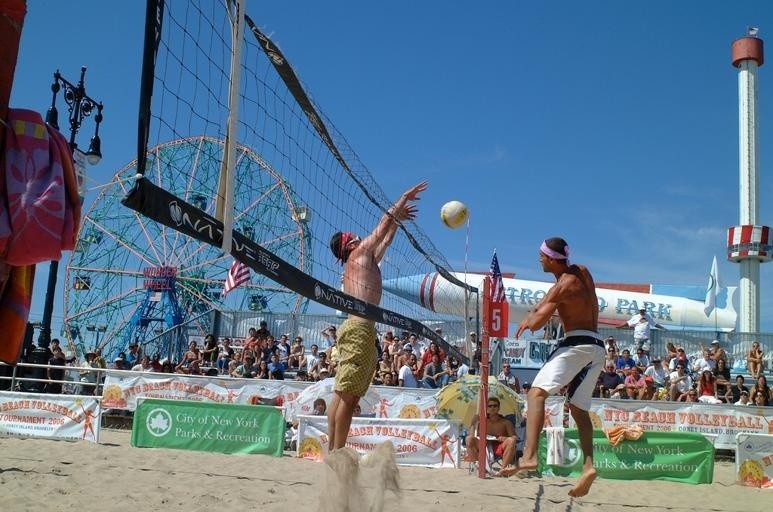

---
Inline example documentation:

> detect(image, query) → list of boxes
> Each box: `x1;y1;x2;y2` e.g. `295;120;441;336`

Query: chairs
468;415;517;476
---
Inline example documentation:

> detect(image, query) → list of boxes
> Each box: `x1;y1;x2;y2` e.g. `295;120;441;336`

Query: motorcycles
676;368;700;402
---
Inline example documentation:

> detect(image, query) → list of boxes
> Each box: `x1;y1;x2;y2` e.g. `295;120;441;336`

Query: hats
435;327;442;332
83;351;97;360
469;331;477;336
650;356;662;363
711;339;720;344
522;380;531;388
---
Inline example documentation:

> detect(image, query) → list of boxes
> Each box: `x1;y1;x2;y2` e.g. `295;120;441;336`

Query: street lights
26;66;103;392
87;325;105;347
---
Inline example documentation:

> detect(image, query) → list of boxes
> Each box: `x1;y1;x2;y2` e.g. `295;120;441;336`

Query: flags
702;255;722;319
218;257;250;299
488;252;507;304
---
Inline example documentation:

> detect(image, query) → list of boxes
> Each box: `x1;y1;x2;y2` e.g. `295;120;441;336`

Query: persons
498;363;569;396
464;396;518;473
615;306;664;357
45;338;172;416
175;320;337;382
372;327;482;389
328;180;429;451
592;336;773;407
497;236;607;498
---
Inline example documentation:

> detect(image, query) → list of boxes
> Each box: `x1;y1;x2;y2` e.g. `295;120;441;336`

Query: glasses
677;350;684;353
739;393;747;396
344;234;362;251
393;340;398;342
319;356;325;358
676;366;684;370
608;349;614;352
487;404;498;408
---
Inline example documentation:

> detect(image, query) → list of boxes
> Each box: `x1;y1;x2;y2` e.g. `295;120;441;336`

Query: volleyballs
441;201;468;227
